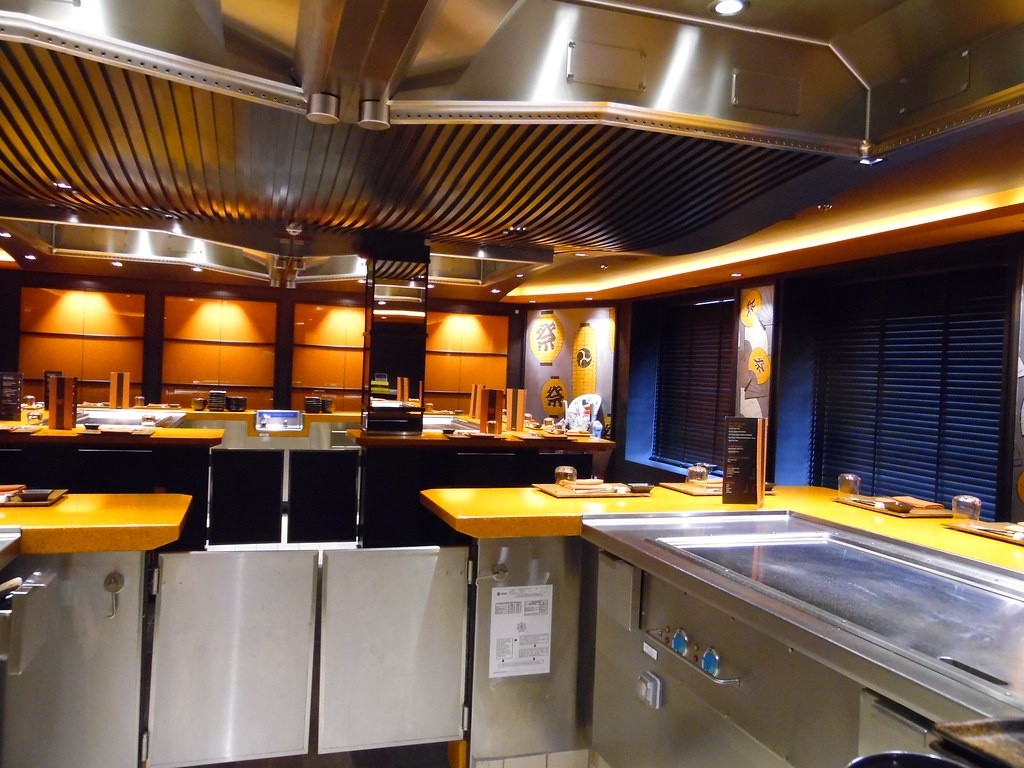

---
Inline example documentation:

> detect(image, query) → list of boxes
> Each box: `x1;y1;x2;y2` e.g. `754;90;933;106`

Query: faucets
260;418;267;428
283;420;287;427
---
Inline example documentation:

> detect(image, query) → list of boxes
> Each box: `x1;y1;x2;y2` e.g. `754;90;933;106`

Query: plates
207;390;226;411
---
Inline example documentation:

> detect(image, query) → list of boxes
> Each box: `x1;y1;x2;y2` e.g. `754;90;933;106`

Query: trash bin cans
846;750;966;768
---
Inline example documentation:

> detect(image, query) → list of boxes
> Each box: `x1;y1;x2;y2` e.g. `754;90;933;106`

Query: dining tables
0;404;1024;768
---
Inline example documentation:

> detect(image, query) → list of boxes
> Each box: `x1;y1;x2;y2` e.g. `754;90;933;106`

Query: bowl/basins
168;404;180;408
303;395;335;413
84;424;100;429
881;500;913;513
441;429;455;434
102;402;109;406
15;488;54;501
454;410;464;414
226;395;248;412
765;481;777;492
627;482;655;493
533;423;542;428
190;397;207;410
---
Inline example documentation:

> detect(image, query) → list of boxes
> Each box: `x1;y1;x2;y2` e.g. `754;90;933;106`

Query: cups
22;394;146;428
951;495;982;531
554;466;577;495
423;402;555;436
838;473;861;503
686;466;708;493
